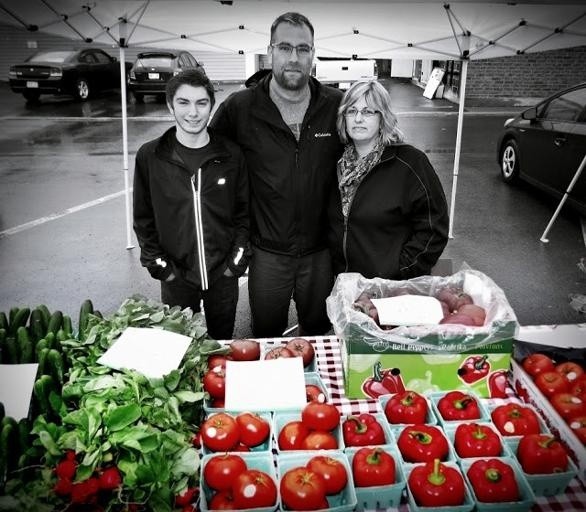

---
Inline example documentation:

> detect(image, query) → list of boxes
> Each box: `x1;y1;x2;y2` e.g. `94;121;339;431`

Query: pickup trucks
312;55;381;91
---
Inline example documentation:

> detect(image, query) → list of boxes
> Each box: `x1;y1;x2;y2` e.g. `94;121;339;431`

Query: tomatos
523;353;586;446
200;338;347;510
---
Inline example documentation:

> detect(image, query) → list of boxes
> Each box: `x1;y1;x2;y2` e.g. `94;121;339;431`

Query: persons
326;78;450;281
131;69;252;338
206;11;345;337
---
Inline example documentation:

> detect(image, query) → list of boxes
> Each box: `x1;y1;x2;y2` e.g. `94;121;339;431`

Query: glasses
341;104;383;119
269;40;316;58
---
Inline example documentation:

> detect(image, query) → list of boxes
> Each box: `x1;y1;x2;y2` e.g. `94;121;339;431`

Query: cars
495;80;585;226
126;46;208;103
7;45;134;101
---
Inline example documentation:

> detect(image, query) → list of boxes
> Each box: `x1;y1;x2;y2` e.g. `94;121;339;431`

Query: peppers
343;354;567;506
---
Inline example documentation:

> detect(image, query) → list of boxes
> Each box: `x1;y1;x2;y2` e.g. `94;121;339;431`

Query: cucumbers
0;299;101;495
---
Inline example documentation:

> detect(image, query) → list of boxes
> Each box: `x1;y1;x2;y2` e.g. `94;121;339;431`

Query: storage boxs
334;270;516;402
506;327;585;487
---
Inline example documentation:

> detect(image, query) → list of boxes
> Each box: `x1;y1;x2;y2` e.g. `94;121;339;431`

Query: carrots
52;433;200;512
